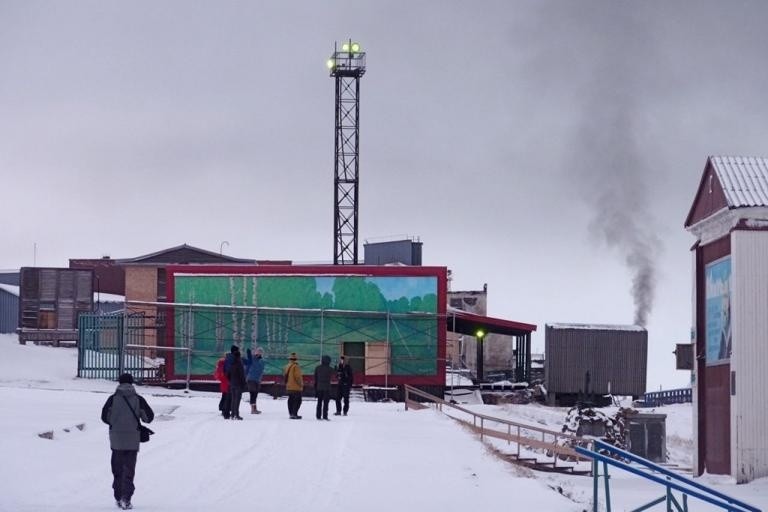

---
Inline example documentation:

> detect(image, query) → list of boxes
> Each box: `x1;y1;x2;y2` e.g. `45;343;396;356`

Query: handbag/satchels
138;423;155;443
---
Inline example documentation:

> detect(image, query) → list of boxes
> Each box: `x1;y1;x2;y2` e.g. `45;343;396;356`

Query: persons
282;351;304;420
214;350;230;413
100;371;155;511
221;345;238;419
229;350;246;420
244;344;265;414
333;353;352;416
312;354;332;420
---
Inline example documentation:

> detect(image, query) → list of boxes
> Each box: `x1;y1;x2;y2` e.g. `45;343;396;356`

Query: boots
250;403;262;414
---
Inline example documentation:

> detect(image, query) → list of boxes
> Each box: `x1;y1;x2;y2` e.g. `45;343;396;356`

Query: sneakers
288;412;348;420
115;499;135;509
223;413;243;420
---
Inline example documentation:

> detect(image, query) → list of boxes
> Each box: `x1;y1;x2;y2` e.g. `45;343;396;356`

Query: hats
288;353;299;360
118;372;134;384
255;346;265;354
